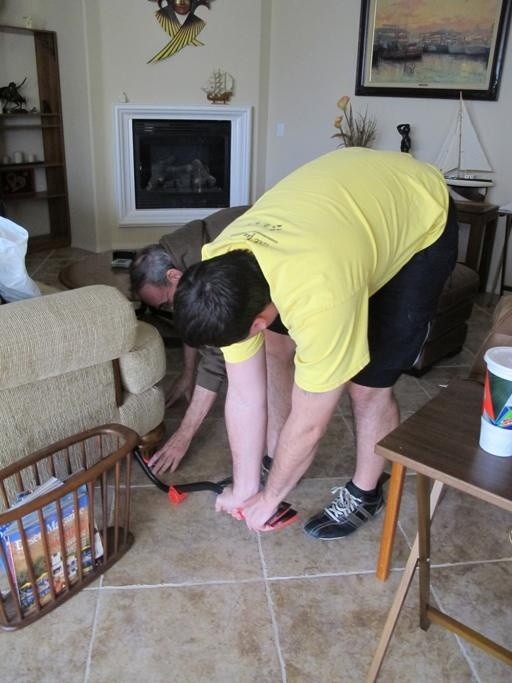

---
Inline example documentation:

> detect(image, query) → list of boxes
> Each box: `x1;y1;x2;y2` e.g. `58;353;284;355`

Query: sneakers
302;472;391;540
216;455;272;490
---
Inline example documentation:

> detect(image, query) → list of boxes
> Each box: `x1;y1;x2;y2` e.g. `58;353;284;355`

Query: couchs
0;285;167;513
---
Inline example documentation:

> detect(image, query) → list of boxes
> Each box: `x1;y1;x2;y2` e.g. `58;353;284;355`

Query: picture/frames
355;1;512;101
115;104;253;228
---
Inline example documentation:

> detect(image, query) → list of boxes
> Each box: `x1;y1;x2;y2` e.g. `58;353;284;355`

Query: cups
478;346;512;458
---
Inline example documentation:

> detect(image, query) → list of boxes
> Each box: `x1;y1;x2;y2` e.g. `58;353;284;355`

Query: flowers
330;95;376;148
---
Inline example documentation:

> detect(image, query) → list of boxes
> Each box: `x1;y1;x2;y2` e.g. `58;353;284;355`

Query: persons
173;145;459;541
126;201;294;492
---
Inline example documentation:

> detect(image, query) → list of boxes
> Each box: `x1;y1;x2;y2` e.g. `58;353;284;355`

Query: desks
498;202;512;297
58;249;142;320
364;377;511;683
456;204;498;294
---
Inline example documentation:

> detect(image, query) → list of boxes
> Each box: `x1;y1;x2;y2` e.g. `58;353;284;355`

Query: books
498;202;512;214
0;467;105;617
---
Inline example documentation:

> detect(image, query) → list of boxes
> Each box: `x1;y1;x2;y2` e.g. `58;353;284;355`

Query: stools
404;264;479;378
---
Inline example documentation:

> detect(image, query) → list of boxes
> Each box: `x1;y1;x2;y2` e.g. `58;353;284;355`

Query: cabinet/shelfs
0;25;71;256
